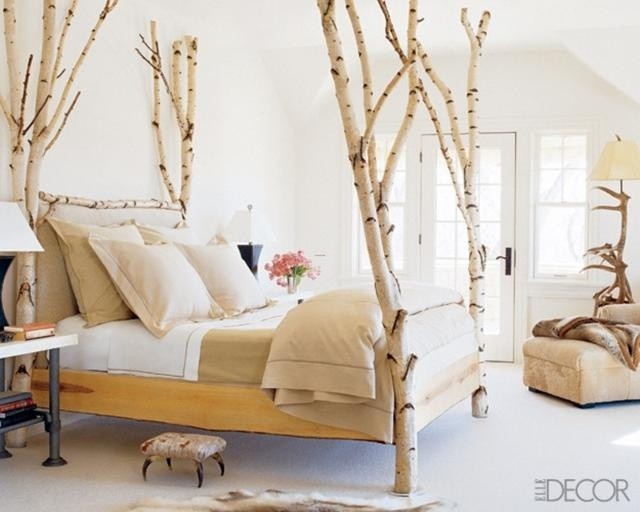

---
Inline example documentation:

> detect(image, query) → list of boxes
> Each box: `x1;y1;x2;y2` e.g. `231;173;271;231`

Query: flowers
263;250;320;295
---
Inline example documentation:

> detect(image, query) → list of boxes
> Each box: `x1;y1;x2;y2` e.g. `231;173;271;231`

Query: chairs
521;304;640;406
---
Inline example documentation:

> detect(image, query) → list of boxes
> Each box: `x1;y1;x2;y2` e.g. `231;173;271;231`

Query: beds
0;0;490;497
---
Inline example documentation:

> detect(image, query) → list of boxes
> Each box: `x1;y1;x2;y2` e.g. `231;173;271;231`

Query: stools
140;433;227;488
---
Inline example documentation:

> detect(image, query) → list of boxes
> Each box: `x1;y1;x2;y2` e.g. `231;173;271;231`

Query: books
0;410;37;429
4;322;57;332
0;404;37;418
0;391;32;406
12;327;55;341
0;398;34;412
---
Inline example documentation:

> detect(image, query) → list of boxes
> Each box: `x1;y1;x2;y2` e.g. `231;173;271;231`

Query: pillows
45;214;275;339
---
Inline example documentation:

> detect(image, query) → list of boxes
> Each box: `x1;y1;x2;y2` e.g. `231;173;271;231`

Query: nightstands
0;332;78;468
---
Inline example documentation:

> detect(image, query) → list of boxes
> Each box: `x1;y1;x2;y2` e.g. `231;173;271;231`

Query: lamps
586;133;640;199
0;200;45;252
